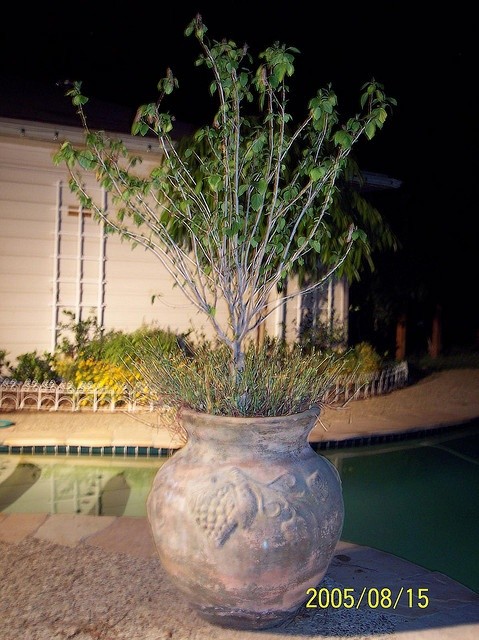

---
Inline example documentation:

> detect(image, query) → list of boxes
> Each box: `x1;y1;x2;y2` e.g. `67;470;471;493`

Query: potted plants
51;14;398;630
